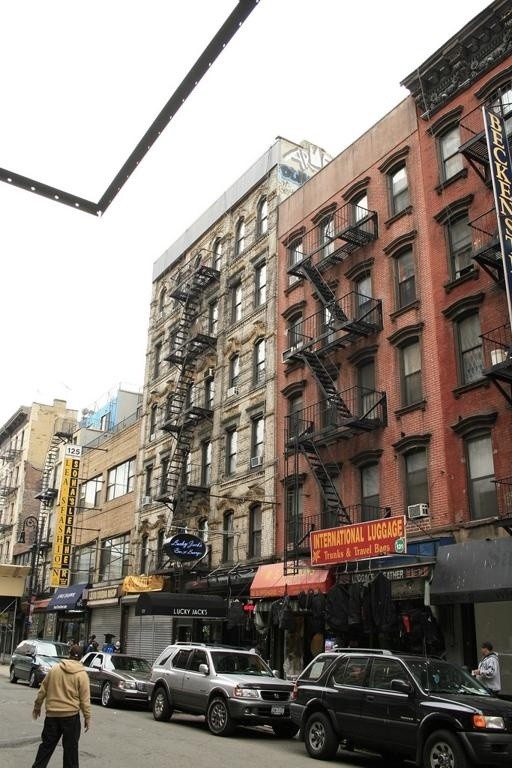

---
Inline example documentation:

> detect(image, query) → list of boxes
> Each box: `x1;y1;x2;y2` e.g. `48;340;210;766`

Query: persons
283;651;294;683
238;657;261;673
31;643;91;768
247;638;262;673
340;738;355;751
67;631;122;655
471;640;503;698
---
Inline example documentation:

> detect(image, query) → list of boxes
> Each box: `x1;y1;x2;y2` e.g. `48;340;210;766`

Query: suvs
290;648;512;768
147;641;301;739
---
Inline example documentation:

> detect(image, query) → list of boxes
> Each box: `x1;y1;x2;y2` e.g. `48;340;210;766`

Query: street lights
17;515;39;639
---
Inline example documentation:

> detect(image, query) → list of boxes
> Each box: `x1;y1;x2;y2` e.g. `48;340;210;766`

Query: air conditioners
226;386;238;397
150;433;156;440
140;496;153;505
407;503;428;518
249;456;263;467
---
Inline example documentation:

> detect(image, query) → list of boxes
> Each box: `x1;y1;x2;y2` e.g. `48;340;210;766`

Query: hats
70;645;83;657
481;641;493;650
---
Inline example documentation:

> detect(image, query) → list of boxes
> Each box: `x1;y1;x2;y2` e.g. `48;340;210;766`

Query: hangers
297;568;389;597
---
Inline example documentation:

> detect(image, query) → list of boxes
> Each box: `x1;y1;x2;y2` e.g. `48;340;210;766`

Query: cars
8;640;77;687
79;652;153;708
